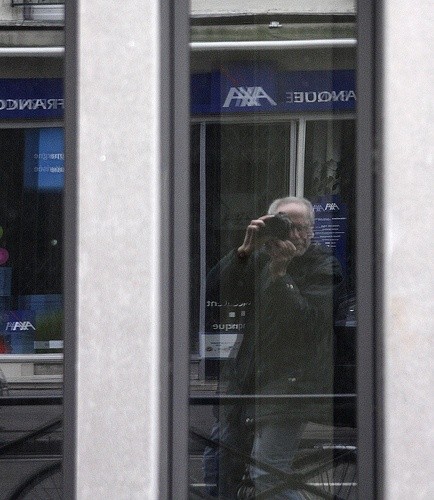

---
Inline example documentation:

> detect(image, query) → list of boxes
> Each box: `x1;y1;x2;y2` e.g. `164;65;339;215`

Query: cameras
262;210;292;240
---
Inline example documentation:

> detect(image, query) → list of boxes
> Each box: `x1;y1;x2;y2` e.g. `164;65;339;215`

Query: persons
205;195;336;500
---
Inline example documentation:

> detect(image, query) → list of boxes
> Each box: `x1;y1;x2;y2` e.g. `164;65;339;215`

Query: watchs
237;248;249;261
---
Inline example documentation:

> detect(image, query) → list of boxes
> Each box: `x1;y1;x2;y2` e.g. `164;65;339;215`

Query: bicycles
4;442;359;500
